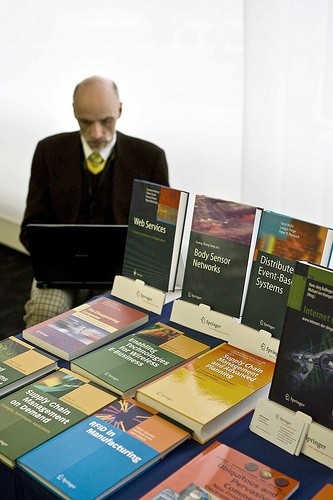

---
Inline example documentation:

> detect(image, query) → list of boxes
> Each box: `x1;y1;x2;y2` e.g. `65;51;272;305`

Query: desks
0;293;333;500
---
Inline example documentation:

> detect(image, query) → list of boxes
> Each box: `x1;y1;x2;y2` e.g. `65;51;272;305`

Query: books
0;179;333;500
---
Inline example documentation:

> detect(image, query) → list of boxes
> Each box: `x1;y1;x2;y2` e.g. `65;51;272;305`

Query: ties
89;152;103;168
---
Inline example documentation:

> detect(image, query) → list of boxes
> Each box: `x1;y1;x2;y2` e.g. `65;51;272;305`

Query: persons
19;75;171;328
